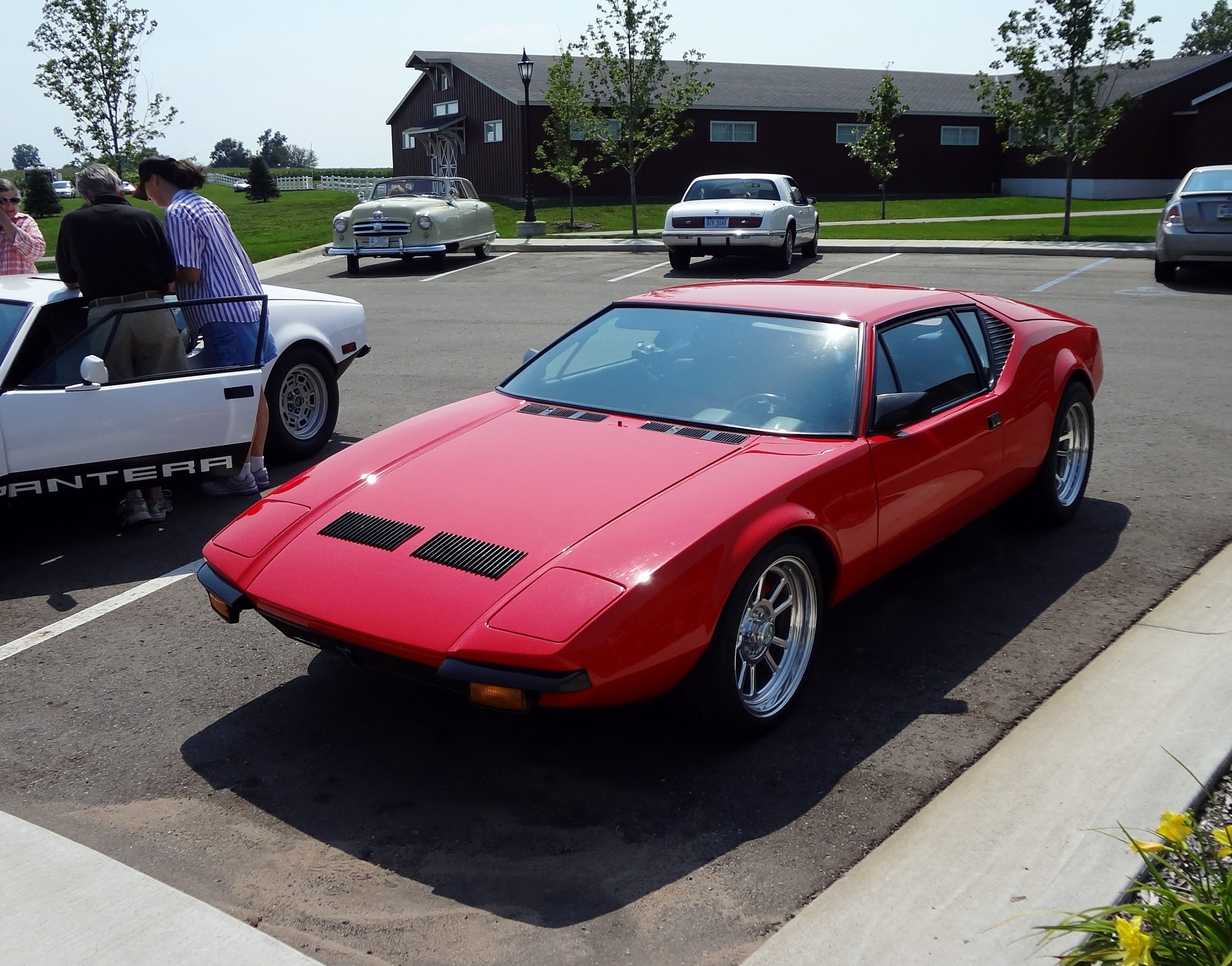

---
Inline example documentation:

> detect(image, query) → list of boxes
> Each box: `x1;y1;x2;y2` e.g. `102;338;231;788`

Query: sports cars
196;279;1104;754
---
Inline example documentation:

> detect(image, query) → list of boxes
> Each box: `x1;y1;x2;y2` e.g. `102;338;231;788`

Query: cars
0;271;374;508
323;176;498;272
1155;164;1232;282
51;180;76;199
233;179;251;192
661;173;820;270
121;181;136;194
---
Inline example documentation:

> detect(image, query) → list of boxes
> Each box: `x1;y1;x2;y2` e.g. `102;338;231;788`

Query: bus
24;163;63;183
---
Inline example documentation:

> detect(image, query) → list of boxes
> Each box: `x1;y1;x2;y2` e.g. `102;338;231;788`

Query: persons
56;163;190;525
389;184;405;197
0;178;46;276
132;158;280;491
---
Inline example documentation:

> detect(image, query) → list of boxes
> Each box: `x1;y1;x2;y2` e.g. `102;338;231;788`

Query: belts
88;291;164;310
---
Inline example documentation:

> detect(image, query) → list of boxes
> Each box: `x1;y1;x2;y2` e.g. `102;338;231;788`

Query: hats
130;157;165;201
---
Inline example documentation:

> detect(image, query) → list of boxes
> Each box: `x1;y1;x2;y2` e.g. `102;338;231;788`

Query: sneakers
150;490;173;521
118;499;152;523
253;467;270;487
202;473;258;494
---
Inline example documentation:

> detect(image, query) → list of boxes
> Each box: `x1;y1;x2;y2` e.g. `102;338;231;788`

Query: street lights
516;47;547;237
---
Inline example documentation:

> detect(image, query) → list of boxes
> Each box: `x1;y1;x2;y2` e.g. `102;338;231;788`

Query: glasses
0;197;20;205
